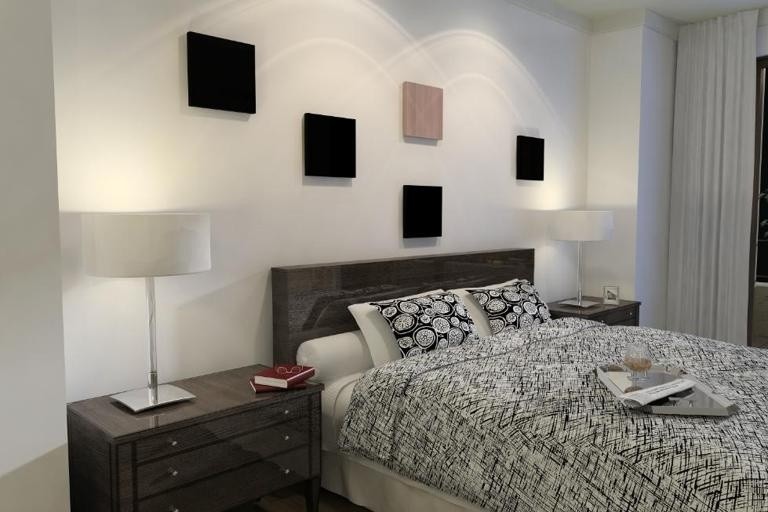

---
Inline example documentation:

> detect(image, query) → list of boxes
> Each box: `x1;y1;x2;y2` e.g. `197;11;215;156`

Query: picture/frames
603;285;620;307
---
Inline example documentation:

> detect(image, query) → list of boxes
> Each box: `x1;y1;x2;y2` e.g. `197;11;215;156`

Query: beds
269;246;768;512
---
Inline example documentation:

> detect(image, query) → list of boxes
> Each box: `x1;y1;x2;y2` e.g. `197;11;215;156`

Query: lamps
78;211;212;413
549;209;611;309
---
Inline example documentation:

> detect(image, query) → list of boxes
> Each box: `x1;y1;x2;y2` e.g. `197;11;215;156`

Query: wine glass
623;341;653;394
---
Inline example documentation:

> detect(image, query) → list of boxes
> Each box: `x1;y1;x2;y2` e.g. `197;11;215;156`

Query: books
249;363;315;393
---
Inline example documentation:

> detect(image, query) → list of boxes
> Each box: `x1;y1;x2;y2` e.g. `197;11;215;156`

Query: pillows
348;278;557;368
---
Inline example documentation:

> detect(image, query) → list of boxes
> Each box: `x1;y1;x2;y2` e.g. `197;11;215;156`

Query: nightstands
65;363;327;511
545;294;642;326
